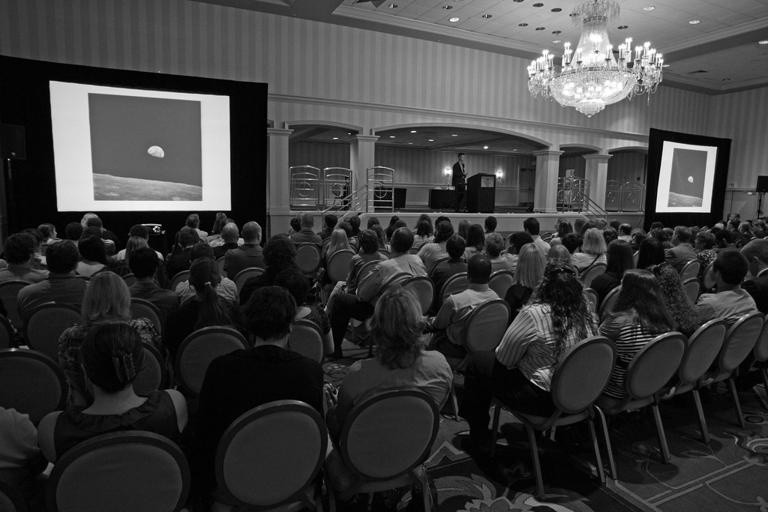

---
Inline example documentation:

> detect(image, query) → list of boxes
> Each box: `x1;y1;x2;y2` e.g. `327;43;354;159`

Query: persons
451;151;469;211
414;212;766;456
2;212;455;510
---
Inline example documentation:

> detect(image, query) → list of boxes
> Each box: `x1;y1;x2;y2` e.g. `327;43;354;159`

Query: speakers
756;176;768;192
0;123;27;160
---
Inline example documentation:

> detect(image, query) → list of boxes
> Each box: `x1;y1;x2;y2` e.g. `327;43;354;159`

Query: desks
371;186;407;212
427;188;470;210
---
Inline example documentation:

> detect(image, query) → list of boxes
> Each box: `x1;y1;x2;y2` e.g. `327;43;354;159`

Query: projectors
138;223;162;235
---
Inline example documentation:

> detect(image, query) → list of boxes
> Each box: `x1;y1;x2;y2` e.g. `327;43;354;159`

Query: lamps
526;1;669;119
